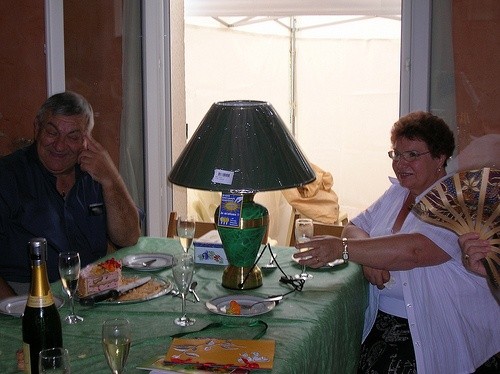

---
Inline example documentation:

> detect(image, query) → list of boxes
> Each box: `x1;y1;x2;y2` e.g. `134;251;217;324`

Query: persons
294;111;500;374
0;91;140;300
457;233;500;374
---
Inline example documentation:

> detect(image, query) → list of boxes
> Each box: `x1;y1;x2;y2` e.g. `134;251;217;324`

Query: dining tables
0;236;370;374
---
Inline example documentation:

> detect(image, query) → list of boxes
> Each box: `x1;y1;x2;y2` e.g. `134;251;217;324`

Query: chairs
289;214;344;249
167;211;268;247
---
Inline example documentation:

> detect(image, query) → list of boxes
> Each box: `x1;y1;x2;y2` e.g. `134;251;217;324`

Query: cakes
76;257;122;298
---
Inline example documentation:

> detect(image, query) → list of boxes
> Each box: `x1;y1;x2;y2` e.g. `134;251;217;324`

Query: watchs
342;238;349;264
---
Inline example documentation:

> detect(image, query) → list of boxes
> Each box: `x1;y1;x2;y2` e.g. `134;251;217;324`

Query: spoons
170;290;196;303
239;296;283;308
189;284;200;302
120;259;157;269
261;253;277;270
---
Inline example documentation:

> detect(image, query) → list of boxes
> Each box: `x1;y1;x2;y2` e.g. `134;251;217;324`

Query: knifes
78;276;151;307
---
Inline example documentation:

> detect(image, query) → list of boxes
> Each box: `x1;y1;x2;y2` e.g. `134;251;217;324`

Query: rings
316;257;320;262
464;254;469;259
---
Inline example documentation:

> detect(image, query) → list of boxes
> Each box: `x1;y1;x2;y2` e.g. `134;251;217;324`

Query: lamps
166;100;317;291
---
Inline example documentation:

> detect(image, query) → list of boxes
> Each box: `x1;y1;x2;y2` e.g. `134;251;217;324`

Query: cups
39;347;72;374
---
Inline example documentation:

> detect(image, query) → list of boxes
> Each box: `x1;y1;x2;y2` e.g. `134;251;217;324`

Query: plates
0;294;65;318
292;251;345;269
62;273;174;304
206;295;276;317
119;253;175;272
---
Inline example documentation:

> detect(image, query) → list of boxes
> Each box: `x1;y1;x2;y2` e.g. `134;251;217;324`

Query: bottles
22;236;62;374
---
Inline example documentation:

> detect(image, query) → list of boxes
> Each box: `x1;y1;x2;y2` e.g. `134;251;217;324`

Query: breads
227;299;241;314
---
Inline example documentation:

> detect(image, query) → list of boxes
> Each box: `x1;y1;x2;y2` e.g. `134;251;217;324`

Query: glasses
388;150;432;161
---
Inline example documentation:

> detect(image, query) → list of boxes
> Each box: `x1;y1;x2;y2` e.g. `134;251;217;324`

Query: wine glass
295;218;314;281
171;253;196;327
102;318;131;374
58;251;83;324
177;216;196;275
